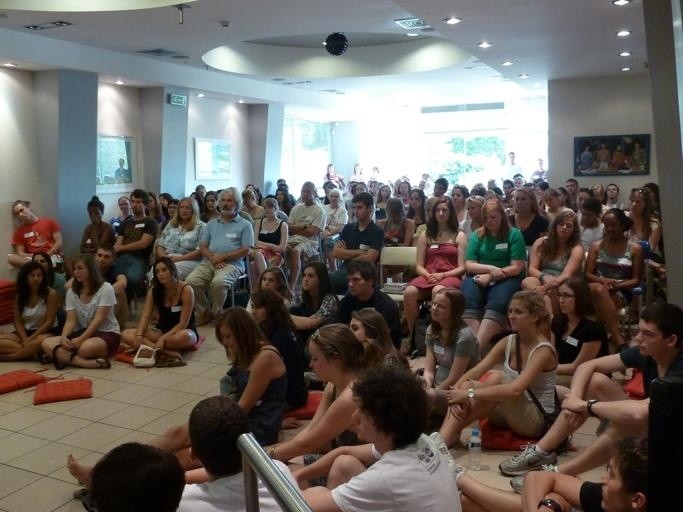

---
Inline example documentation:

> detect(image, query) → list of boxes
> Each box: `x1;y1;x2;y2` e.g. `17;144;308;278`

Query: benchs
125;283;152;314
229;246;327;307
380;247;664;340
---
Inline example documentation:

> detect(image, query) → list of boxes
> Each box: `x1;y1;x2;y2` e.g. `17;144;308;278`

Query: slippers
95;358;111;370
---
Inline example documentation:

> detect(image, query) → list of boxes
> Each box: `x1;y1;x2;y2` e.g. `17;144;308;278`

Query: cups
386;278;392;284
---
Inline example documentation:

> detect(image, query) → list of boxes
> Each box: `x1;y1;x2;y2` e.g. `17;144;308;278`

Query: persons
40;253;123;371
0;260;63;364
32;252;68;330
62;151;682;510
578;140;647;174
6;200;62;273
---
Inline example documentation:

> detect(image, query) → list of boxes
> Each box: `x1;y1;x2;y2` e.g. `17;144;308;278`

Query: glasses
631;188;649;193
556;292;575;298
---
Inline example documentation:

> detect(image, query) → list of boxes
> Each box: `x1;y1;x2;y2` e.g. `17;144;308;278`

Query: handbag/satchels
546;404;571;452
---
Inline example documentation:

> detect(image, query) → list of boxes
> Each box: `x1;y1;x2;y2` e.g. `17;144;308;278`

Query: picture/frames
193;136;230;182
96;134;137;195
573;134;650;176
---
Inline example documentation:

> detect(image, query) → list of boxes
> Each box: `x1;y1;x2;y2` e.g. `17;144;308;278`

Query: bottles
467;430;482;471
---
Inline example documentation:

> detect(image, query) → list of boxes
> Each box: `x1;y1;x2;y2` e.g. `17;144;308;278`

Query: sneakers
429;432;463;479
499;442;557;478
510;464;558;495
